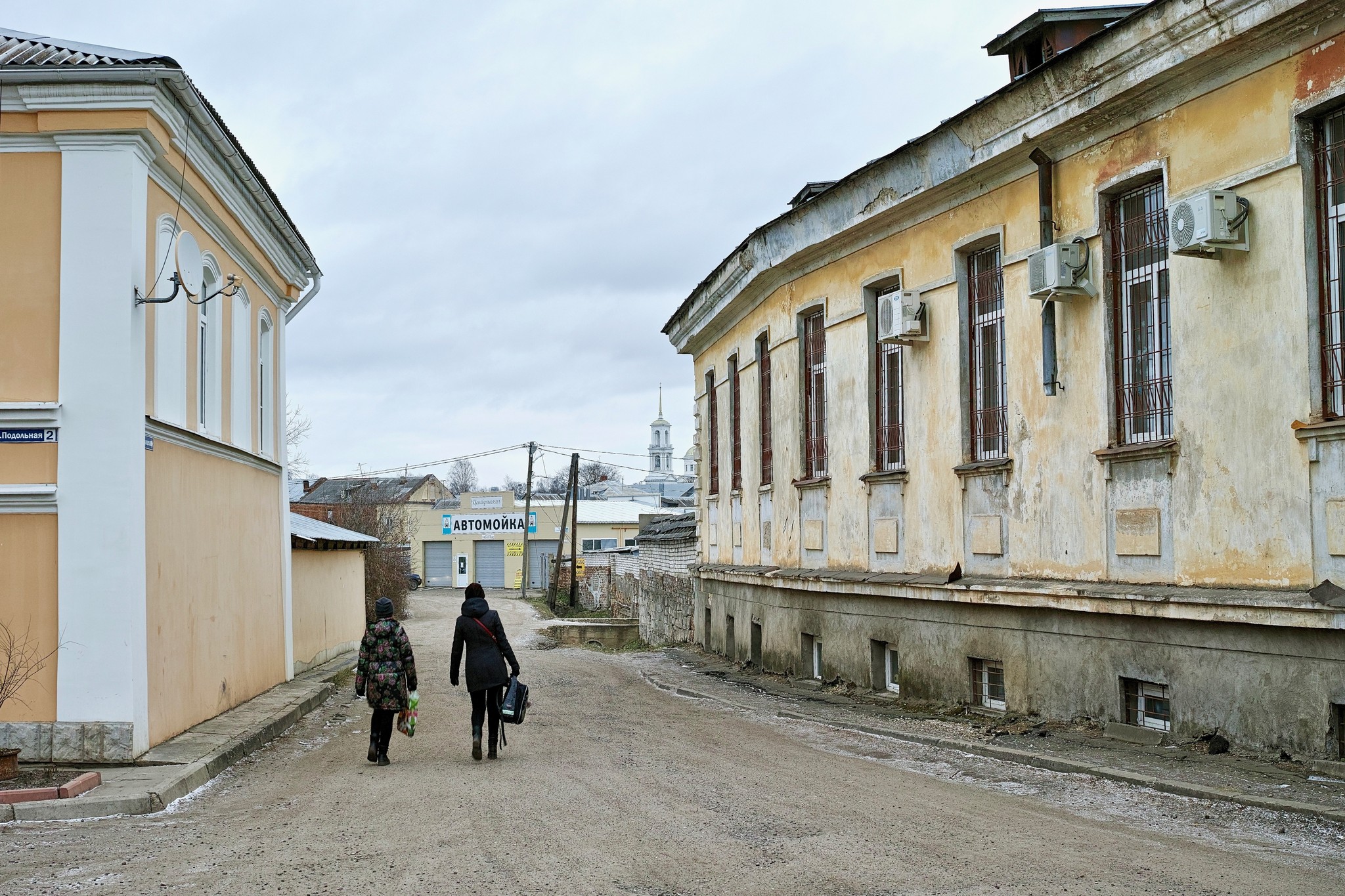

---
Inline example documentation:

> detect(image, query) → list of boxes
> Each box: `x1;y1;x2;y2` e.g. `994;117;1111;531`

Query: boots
488;733;497;760
368;734;380;762
472;725;482;760
378;741;390;766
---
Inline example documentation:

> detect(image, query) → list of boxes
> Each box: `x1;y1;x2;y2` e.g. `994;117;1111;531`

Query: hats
375;597;394;619
465;583;485;600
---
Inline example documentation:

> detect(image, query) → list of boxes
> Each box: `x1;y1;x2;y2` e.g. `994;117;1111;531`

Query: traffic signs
576;558;585;576
507;545;523;554
513;569;522;590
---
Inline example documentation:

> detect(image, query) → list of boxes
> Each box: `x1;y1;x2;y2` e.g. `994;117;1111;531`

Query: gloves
510;670;520;678
450;669;459;686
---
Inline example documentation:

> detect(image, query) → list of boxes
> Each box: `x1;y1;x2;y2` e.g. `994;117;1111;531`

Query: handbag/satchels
397;689;419;737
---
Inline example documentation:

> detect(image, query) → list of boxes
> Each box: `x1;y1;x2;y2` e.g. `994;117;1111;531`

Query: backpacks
496;676;528;725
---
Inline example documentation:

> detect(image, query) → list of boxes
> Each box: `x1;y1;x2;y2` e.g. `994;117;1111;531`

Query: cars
402;570;422;590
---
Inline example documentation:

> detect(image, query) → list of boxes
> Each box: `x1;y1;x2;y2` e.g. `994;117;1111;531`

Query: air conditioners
1168;190;1238;252
1027;243;1081;296
878;290;921;340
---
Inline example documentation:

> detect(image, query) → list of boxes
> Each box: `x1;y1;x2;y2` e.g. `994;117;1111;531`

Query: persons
450;583;520;760
355;597;418;766
